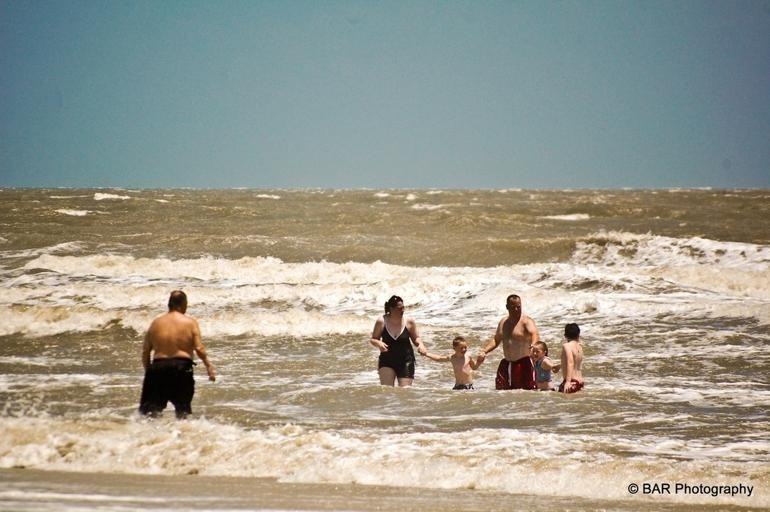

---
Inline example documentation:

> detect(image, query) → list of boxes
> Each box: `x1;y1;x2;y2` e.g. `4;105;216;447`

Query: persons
370;294;426;387
527;340;555;392
550;322;585;393
480;293;539;390
417;335;486;390
138;289;216;418
